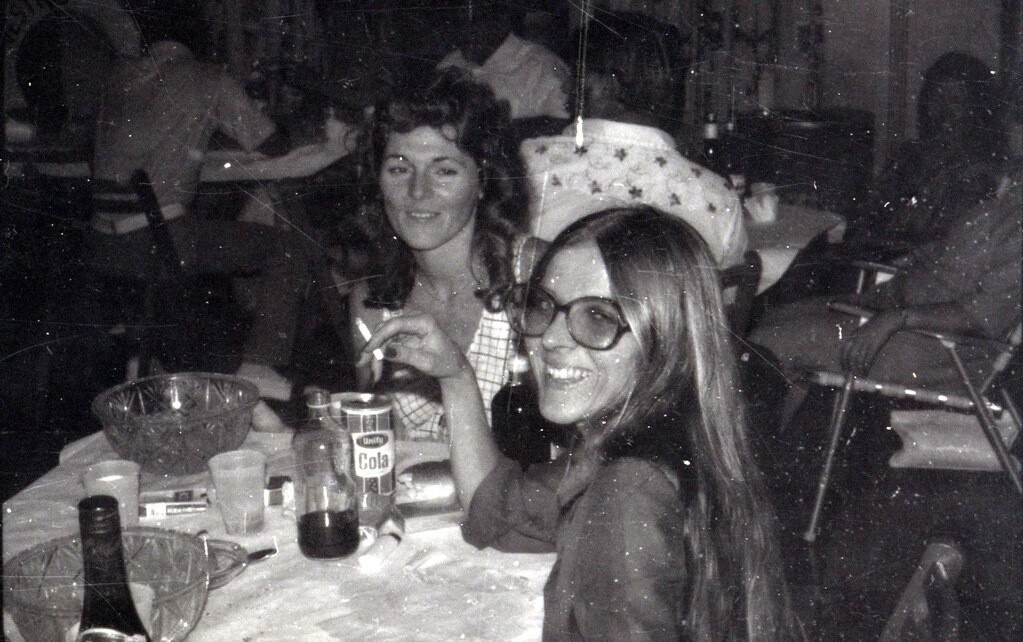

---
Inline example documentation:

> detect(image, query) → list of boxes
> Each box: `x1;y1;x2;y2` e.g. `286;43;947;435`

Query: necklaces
414;270;481;302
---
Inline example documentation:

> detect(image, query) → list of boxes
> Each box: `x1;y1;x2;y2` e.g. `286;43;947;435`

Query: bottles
696;113;721;177
76;496;156;642
292;390;361;562
719;123;745;201
489;356;552;472
245;59;269;110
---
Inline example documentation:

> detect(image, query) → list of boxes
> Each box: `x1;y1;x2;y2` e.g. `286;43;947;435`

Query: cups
127;581;153;634
64;621;81;642
209;450;267;537
751;183;775;202
81;459;140;526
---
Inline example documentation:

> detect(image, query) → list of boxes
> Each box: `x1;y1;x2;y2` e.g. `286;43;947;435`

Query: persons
354;204;795;642
513;9;748;304
425;0;571;123
313;65;556;429
743;79;1023;435
87;1;308;403
850;50;1006;287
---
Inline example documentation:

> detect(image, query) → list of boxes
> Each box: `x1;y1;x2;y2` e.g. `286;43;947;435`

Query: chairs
801;262;1023;543
20;161;248;381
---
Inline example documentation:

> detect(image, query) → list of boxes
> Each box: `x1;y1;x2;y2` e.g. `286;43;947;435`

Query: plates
202;538;247;590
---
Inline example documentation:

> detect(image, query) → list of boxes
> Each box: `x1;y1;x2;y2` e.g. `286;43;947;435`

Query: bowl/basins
3;526;213;642
91;371;259;477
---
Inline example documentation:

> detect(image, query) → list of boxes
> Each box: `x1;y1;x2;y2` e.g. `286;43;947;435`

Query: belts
90;202;184;235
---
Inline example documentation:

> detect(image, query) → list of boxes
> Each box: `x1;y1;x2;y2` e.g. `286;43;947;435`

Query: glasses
502;283;632;350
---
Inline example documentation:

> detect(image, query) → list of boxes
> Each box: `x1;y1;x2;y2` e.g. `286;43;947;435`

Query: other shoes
233;359;294;402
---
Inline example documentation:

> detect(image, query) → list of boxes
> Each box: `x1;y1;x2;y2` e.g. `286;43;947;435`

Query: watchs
893;299;913;330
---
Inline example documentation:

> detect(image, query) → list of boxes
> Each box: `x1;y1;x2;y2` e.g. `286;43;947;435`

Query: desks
1;432;556;641
201;106;377;182
746;199;848;295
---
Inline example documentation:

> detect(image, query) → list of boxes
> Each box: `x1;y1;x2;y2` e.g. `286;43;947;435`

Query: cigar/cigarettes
354;318;386;362
